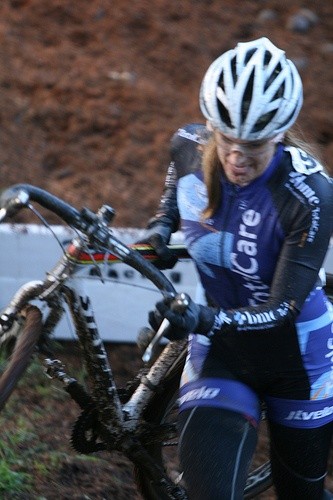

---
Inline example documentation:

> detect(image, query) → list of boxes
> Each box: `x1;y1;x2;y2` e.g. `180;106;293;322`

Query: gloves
148;292;215;342
134;221;178;270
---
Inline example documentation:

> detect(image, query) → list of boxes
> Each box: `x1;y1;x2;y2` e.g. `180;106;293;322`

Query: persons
134;37;333;500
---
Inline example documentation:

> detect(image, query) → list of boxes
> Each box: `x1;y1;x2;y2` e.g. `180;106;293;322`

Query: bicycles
1;183;333;500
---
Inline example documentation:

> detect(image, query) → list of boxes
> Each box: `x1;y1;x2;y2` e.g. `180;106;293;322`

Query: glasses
212;129;280;155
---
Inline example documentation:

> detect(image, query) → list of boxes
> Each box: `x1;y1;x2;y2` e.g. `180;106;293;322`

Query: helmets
199;38;303;142
